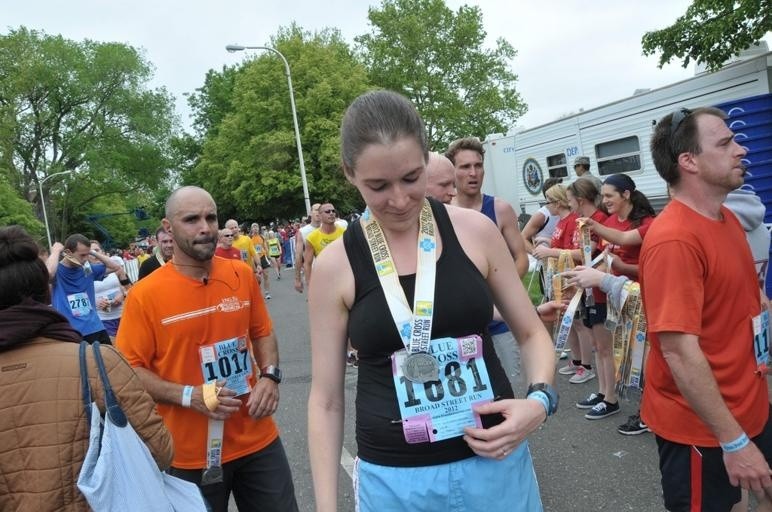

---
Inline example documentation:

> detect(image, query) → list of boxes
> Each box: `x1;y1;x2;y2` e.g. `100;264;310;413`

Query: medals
201;468;224;487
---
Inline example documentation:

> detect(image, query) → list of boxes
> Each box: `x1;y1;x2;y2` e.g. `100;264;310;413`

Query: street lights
224;44;313;222
35;169;69;253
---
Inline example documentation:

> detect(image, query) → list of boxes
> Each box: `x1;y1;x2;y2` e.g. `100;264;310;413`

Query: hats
574;157;590;165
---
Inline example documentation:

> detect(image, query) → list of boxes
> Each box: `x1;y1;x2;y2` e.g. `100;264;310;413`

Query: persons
426;138;657;435
215;203;359;368
722;165;771;402
638;106;772;512
0;225;177;512
39;226;176;347
113;186;298;511
306;88;557;512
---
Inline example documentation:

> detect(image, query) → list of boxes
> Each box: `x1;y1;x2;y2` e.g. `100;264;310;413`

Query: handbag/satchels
76;401;207;512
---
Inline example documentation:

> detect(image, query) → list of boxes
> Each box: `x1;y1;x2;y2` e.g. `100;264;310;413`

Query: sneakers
576;393;620;419
559;361;596;383
618;413;652;434
347;351;359;368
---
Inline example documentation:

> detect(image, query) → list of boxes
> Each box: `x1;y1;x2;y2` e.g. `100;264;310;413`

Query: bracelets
719;433;750;454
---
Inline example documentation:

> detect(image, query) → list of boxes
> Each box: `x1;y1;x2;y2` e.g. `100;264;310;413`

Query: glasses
325;209;335;213
671;107;688;137
